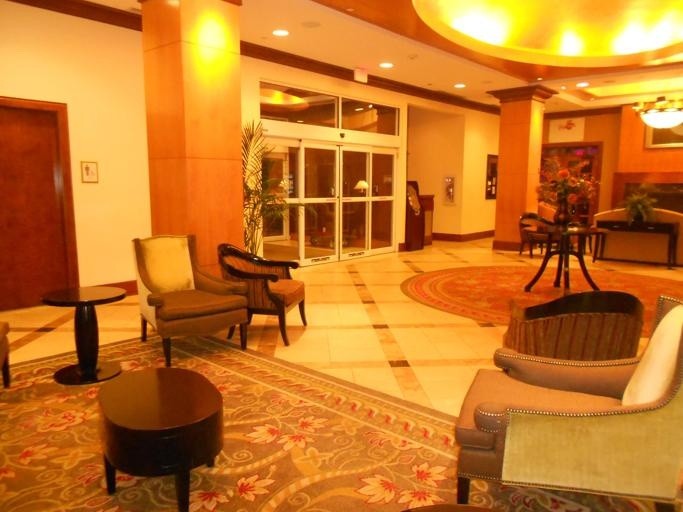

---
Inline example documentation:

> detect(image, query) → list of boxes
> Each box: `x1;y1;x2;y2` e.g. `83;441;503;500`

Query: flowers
535;157;594;206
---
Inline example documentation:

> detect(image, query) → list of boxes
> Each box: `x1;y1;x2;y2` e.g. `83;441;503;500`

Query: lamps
632;97;683;129
353;180;368;191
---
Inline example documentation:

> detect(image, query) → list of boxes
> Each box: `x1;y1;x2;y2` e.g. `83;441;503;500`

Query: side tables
42;287;127;386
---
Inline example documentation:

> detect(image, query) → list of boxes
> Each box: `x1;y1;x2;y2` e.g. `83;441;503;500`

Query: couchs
592;209;683;266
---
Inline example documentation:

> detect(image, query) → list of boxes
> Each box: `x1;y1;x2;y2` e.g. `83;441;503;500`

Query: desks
419;194;434;245
524;226;608;292
592;221;679;269
98;368;223;512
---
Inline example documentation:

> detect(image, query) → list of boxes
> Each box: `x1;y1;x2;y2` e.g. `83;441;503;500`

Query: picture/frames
541;141;602;224
644;124;683;149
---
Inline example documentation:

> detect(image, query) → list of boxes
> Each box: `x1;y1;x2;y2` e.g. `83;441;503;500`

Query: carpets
401;266;683;338
0;335;683;512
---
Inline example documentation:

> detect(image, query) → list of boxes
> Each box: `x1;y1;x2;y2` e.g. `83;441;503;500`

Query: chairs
0;321;10;388
519;212;553;258
503;291;644;360
217;243;307;346
133;234;248;368
456;304;683;512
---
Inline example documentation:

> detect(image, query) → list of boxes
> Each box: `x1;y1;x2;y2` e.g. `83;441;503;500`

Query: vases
553;204;570;224
